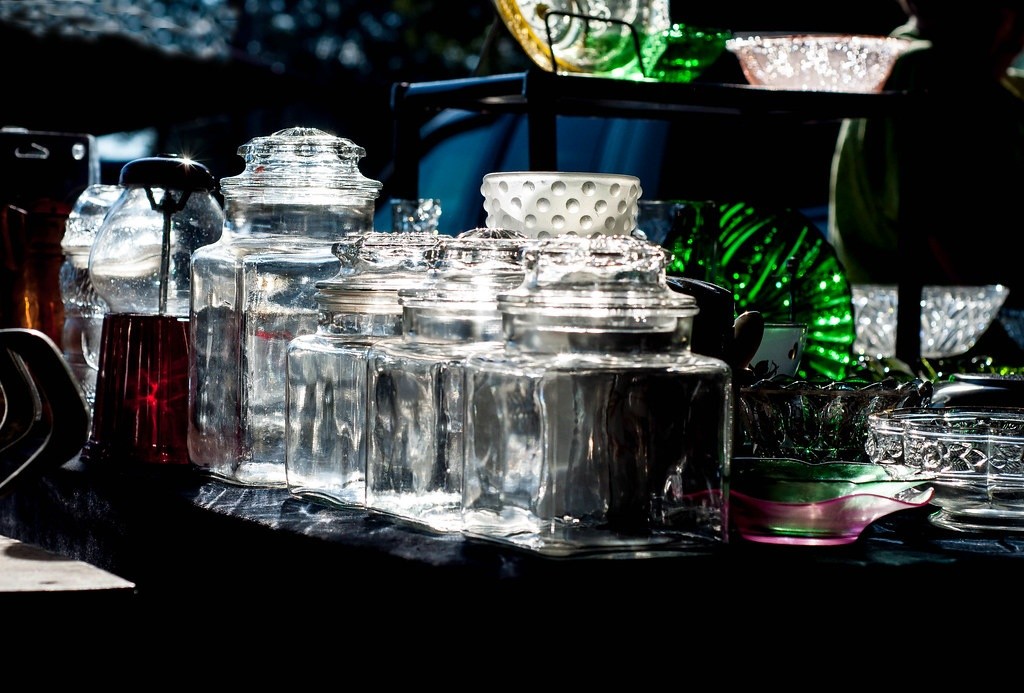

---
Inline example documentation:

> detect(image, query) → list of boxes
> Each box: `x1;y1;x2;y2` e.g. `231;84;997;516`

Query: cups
3;200;70;352
79;311;191;471
638;200;716;280
390;198;442;232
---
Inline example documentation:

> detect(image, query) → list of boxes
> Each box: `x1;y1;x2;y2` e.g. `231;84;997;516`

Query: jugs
88;152;223;313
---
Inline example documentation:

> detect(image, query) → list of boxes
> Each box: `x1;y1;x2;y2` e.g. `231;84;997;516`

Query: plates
495;0;670;77
660;196;855;382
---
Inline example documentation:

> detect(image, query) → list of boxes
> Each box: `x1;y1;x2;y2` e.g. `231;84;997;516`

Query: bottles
188;128;384;531
59;184;126;410
369;228;541;535
283;232;447;509
445;234;730;560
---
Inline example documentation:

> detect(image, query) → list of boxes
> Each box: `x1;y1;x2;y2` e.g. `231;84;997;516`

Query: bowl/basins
725;33;932;94
734;381;934;464
731;457;933;545
854;282;1011;360
865;406;1024;507
746;324;809;380
586;23;732;83
481;171;643;238
